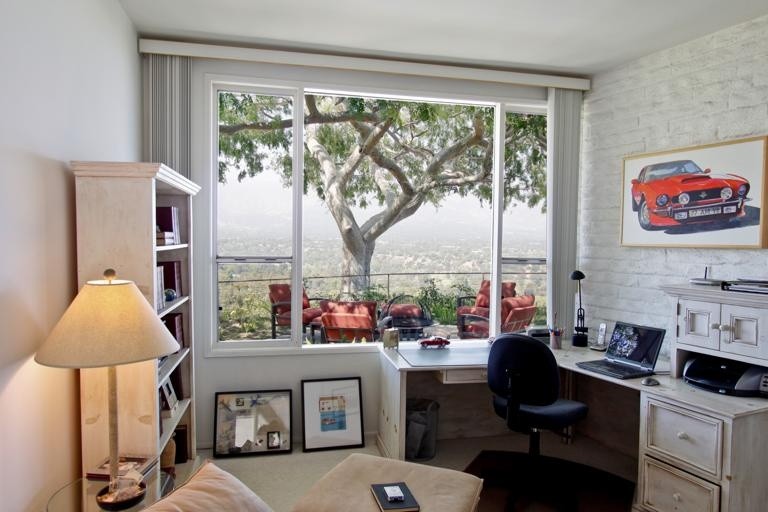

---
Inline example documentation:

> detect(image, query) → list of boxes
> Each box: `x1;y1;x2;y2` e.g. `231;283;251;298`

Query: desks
381;316;441;340
45;460;177;512
310;315;323;342
375;338;677;463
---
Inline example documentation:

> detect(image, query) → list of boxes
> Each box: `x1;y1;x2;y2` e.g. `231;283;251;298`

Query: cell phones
382;484;404;502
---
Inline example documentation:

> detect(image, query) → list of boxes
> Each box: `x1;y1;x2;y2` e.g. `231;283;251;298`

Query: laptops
575;320;666;381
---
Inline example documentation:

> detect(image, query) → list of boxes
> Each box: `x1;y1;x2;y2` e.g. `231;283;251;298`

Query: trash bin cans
406;398;439;462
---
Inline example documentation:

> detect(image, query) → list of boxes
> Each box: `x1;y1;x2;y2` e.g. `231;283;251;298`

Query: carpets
462;442;635;512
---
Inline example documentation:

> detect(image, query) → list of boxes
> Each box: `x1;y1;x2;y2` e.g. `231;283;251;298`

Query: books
156;206;187;498
370;482;420;512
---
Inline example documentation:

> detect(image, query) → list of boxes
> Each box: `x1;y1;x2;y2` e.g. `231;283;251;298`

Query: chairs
458;292;537;338
487;331;590;505
456;280;518;332
267;282;327;341
320;297;381;343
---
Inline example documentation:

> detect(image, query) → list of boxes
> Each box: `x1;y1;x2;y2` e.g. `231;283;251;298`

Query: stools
375;300;424;318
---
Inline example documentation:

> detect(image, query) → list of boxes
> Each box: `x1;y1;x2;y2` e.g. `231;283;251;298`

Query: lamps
568;268;590;347
32;275;181;502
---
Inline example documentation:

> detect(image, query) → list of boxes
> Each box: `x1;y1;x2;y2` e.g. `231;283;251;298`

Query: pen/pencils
548;312;566;349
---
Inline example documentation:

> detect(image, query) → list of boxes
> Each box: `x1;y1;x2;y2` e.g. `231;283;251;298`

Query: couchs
140;459;278;512
289;449;485;512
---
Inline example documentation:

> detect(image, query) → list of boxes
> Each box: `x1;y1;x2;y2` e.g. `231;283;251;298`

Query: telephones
589;323;609;351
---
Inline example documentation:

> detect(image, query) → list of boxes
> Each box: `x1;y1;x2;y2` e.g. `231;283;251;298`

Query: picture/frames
619;135;768;250
213;387;294;460
299;374;366;453
85;445;161;477
160;377;178;409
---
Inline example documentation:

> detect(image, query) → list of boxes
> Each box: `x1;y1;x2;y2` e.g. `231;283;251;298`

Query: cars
631;160;750;231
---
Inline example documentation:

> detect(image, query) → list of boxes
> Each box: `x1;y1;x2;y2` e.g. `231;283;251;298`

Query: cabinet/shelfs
662;281;768;363
634;390;768;512
72;158;201;512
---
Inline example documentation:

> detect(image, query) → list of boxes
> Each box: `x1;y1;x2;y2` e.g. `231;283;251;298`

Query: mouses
641;377;659;386
